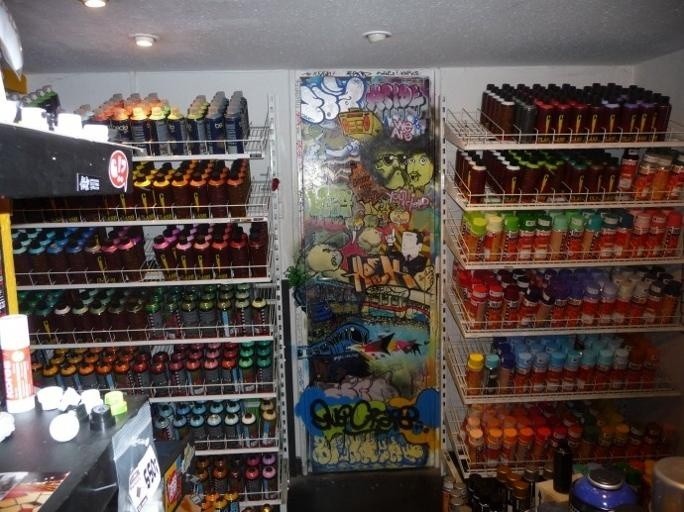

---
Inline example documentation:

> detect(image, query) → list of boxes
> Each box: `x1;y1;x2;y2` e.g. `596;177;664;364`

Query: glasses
375;153;408;164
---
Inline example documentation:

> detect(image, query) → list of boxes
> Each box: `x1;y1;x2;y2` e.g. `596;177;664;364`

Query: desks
0;386;153;511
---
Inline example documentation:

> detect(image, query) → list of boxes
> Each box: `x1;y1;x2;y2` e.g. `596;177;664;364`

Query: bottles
443;84;684;512
0;84;282;512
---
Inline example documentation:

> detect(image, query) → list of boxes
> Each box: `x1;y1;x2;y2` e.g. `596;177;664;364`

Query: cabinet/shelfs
0;82;291;511
2;95;135;204
444;108;684;511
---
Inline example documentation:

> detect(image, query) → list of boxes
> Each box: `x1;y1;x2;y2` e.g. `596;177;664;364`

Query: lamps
82;1;108;9
361;30;391;43
128;32;159;47
